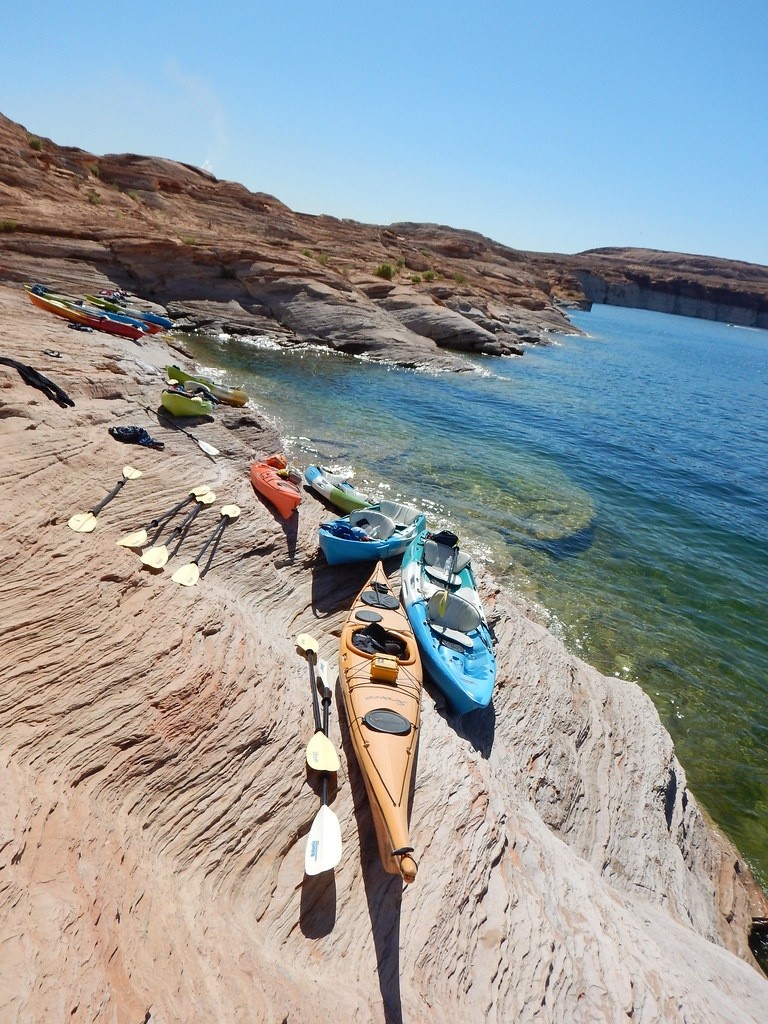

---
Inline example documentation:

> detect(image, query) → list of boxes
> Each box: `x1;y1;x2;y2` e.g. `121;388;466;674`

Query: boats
304;464;380;512
249;453;302;519
401;530;496;715
318;498;427;567
342;560;426;882
164;364;249;407
23;283;175;334
25;289;163;341
160;380;220;417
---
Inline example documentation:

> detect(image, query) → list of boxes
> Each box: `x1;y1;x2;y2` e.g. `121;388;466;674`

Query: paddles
138;491;217;569
294;632;342;773
65;465;143;533
134;396;221;456
438;537;461;619
303;658;343;877
114;485;211;549
46;288;106;308
169;503;242;587
